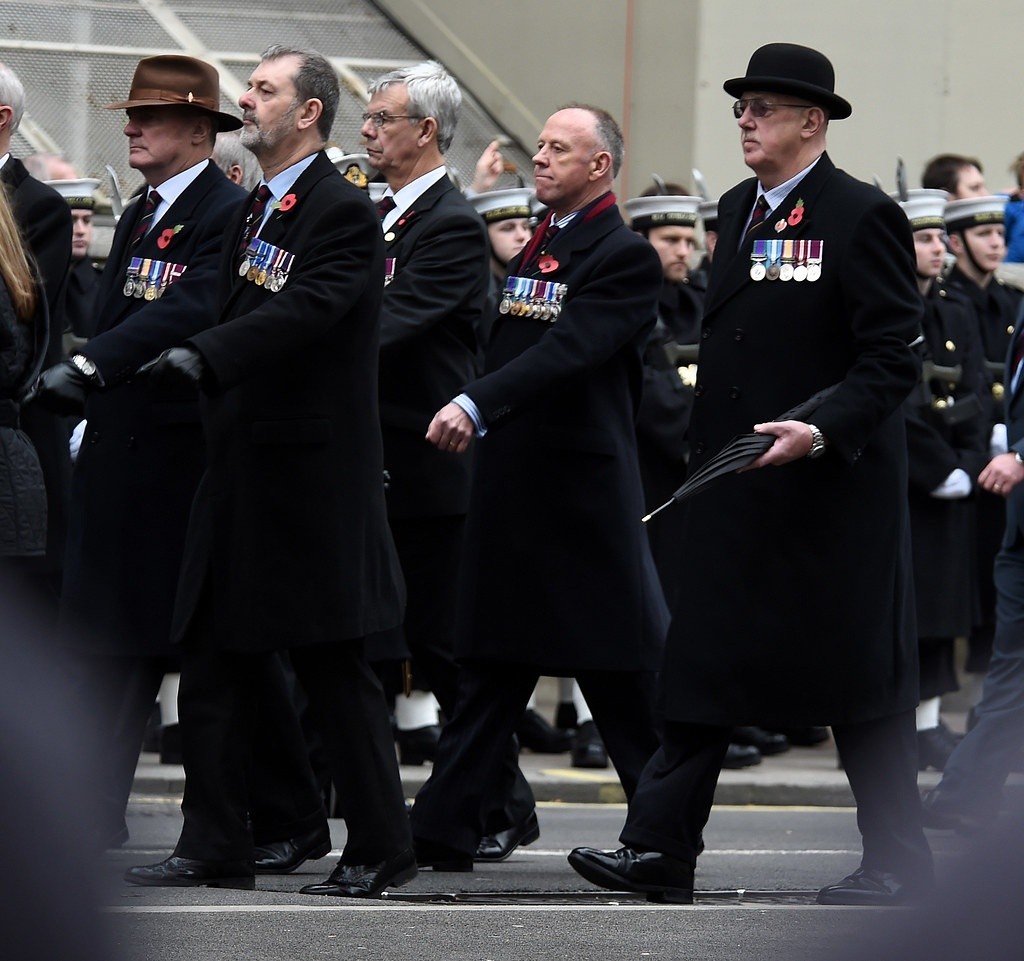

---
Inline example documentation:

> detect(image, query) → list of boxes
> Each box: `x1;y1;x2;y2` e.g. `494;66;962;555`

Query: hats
697;200;720;232
104;54;243;133
623;196;702;232
942;195;1010;236
463;188;533;225
723;42;852;120
39;178;102;211
529;191;548;235
887;189;951;204
367;183;389;204
896;198;947;233
327;154;379;195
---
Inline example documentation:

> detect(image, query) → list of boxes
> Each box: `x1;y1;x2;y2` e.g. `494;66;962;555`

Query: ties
375;196;397;226
741;195;771;252
240;185;273;255
520;225;560;277
127;190;164;256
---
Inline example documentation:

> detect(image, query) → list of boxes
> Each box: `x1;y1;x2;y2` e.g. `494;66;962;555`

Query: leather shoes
569;845;698;905
252;820;332;874
475;811;540;862
300;844;419;896
126;854;256;890
817;866;934;904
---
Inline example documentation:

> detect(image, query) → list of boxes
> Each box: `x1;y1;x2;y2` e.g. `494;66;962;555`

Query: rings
994;484;1001;490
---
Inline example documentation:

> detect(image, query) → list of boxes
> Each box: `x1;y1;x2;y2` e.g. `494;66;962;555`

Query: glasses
360;113;425;127
731;98;813;119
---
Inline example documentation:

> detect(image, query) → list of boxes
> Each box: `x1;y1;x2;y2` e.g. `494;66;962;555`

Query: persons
0;42;1024;903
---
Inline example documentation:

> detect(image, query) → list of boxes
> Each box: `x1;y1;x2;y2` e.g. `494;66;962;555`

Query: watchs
806;424;826;458
72;354;99;384
1014;452;1024;466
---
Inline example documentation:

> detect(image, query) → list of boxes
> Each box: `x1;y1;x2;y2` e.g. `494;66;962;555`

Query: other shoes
722;742;762;769
396;727;441;767
523;710;568;754
554;700;577;752
732;728;787;755
920;795;998;838
570;724;609;768
160;723;190;765
793;727;830;748
